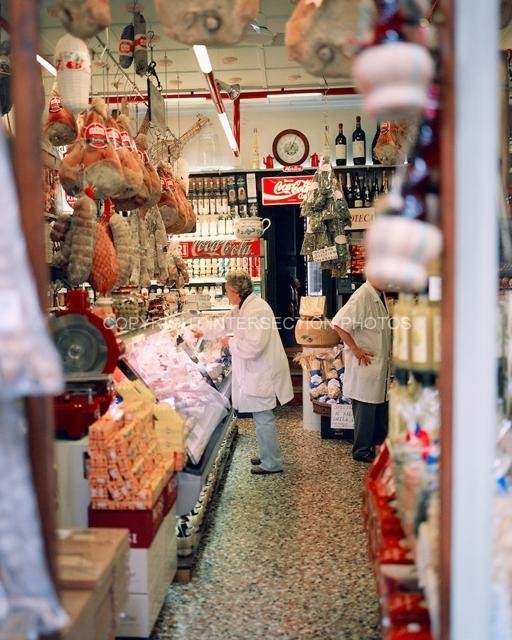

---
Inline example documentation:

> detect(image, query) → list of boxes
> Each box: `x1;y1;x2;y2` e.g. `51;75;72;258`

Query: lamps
192;43;242;158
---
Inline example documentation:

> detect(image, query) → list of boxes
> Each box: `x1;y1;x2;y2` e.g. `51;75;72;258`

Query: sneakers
251;458;262;464
251;466;282;473
354;451;375;463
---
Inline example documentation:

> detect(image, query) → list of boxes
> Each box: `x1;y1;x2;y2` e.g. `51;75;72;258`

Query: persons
328;277;393;464
190;270;297;474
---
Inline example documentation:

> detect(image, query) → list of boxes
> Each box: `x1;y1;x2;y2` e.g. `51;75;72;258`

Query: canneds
183;256;260;278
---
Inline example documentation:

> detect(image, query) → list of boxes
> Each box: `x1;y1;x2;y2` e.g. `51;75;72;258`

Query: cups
234;217;271;240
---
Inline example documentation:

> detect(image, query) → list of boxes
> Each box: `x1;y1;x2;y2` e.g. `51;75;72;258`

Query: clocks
272;129;309;167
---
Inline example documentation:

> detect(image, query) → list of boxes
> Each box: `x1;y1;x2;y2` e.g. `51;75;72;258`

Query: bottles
249;127;260;170
169;175;257;237
55;283;222;331
320;125;330;165
332;115;404;208
385;288;512;453
